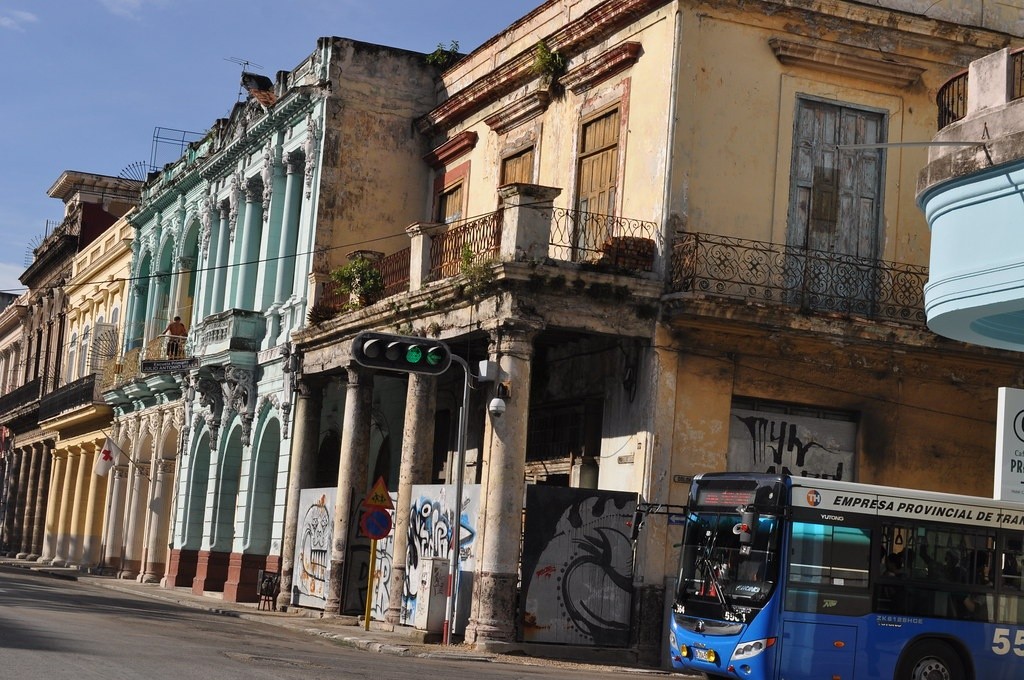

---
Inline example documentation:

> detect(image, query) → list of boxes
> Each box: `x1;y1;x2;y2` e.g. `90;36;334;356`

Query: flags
93;437;123;479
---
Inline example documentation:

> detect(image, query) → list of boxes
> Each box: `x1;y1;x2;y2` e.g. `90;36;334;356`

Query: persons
161;315;188;359
880;541;969;579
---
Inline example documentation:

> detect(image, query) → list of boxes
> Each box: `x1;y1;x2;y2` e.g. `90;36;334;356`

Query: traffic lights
350;328;454;377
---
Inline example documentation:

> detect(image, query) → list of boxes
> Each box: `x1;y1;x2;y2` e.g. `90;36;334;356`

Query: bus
666;469;1024;680
